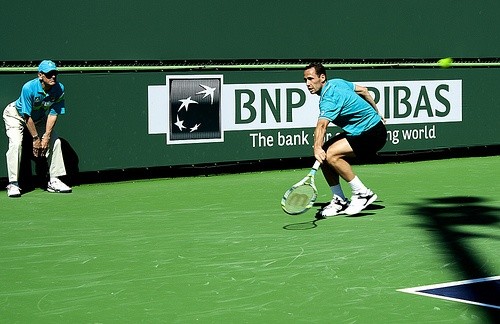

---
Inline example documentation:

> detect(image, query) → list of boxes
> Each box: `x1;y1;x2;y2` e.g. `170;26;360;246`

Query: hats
38;59;59;75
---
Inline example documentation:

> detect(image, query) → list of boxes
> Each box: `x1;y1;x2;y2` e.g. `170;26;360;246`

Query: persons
3;60;72;197
304;63;387;216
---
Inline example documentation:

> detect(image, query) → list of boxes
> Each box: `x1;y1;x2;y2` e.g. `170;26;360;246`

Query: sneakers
47;178;72;192
320;195;348;219
343;188;378;216
7;183;20;196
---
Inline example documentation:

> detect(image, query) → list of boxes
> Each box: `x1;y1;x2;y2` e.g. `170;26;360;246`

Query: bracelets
33;135;38;141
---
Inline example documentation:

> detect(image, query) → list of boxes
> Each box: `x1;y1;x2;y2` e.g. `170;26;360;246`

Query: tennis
438;57;453;68
280;160;321;216
